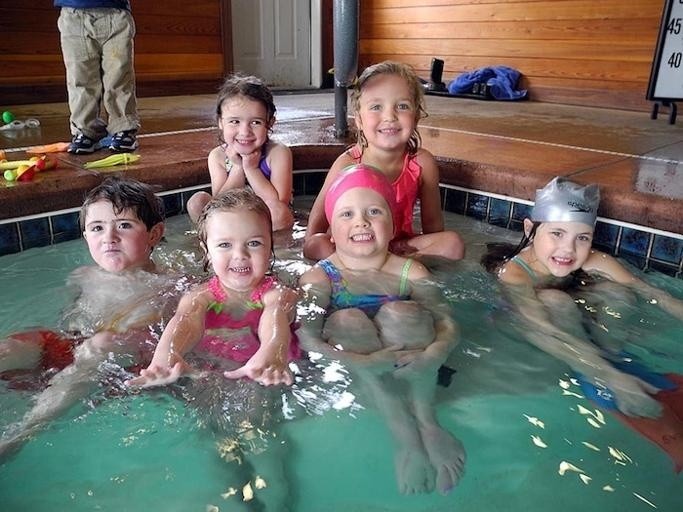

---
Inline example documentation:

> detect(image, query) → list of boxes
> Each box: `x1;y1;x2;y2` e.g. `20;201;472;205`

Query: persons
119;187;308;510
480;175;682;421
181;73;295;239
301;60;466;270
51;0;142;156
293;161;469;497
1;177;187;466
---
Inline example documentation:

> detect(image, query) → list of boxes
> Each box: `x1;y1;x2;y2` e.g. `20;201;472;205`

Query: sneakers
67;131;111;154
108;131;140;153
